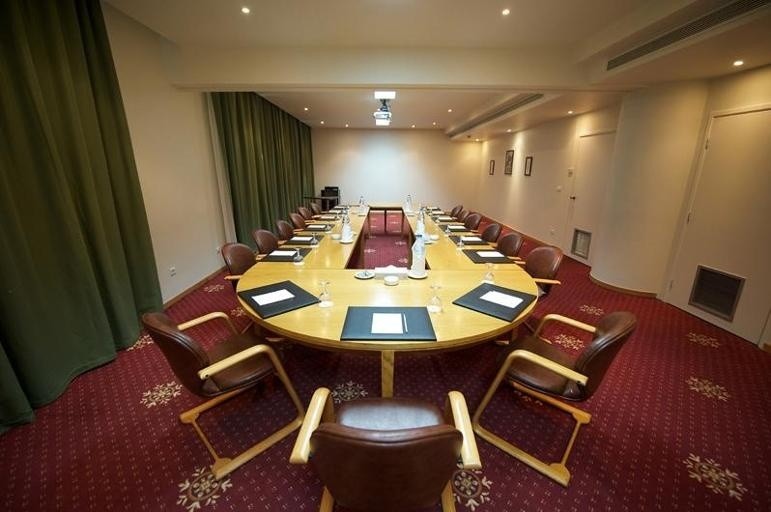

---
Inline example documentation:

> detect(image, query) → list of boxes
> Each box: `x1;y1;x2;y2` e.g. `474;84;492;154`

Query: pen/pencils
404;312;408;333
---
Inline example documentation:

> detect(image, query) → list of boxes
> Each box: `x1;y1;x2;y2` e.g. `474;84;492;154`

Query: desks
304;196;339;210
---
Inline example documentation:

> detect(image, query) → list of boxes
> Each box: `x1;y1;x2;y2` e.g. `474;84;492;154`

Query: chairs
140;311;306;481
472;311;638;487
289;387;482;511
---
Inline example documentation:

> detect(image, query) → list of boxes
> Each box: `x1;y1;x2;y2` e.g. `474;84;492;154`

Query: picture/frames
490;160;494;175
524;157;533;176
504;150;514;175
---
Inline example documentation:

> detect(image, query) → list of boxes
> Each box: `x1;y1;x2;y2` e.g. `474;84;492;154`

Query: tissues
375;264;408;280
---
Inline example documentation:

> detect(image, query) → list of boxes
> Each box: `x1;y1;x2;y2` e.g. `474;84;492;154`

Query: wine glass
318;281;333;307
435;216;496;284
294;211;341;265
427;285;442;313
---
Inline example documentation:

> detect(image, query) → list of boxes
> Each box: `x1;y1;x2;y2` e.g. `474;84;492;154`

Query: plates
355;271;374;279
424;241;433;244
408;272;428;279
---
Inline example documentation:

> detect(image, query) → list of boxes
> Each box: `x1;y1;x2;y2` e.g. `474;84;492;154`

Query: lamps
373;90;395;126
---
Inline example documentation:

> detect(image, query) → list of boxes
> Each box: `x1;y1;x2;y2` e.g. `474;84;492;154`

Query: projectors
373;100;391;118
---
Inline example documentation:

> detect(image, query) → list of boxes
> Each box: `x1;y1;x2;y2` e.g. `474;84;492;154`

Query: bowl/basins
384;276;399;286
430;235;439;241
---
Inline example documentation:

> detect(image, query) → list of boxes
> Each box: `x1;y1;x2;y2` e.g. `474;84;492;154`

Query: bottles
341;195;425;275
